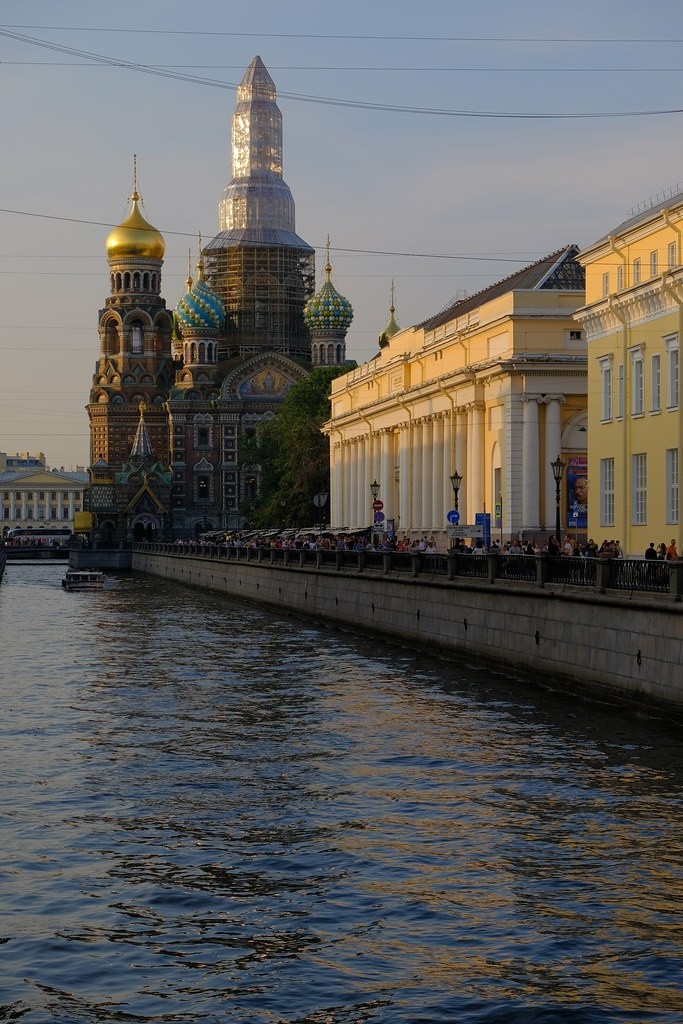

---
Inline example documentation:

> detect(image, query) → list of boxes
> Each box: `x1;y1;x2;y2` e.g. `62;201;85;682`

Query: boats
62;570;107;592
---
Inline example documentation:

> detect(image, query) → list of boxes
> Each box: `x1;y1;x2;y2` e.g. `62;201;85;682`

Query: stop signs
372;500;384;511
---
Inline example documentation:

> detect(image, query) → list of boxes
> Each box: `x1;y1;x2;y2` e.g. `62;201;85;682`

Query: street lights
369;479;381;527
550;455;566;545
449;470;463;525
202;509;209;535
279;496;289;529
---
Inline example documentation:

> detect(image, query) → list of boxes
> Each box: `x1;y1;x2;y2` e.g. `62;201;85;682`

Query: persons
645;543;658;560
666;539;678;561
666;553;673;560
0;516;621;587
656;543;667;560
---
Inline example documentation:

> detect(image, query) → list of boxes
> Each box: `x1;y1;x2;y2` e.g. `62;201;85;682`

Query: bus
6;527;73;550
